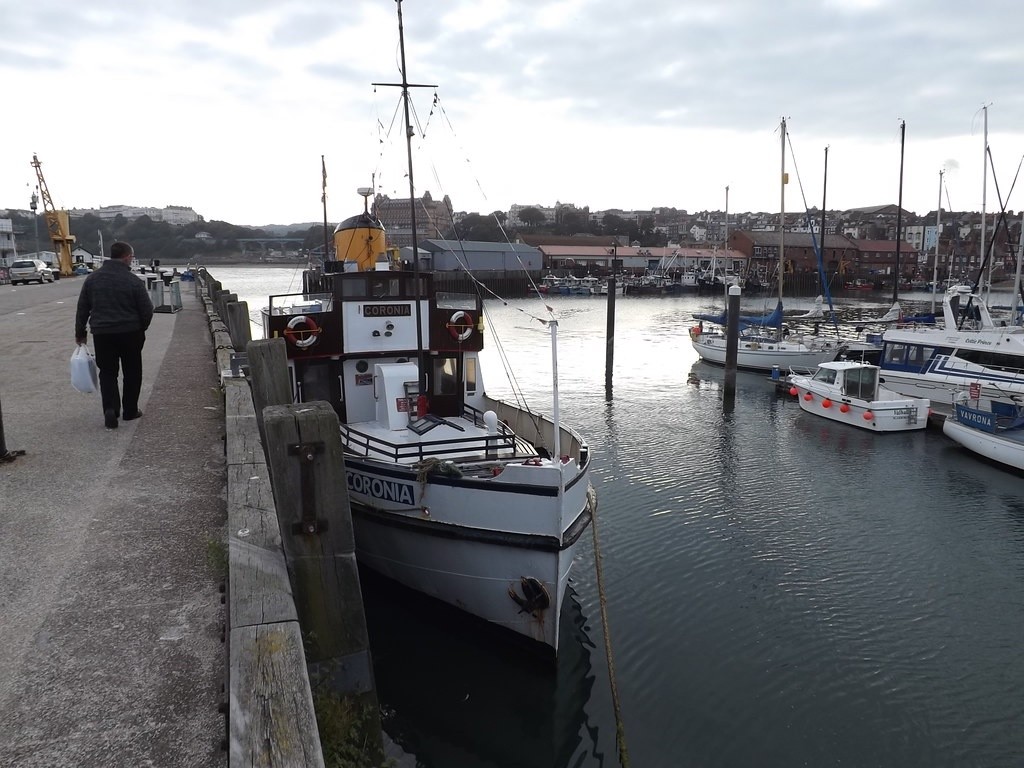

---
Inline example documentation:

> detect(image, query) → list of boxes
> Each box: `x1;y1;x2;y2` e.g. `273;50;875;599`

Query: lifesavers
448;311;473;340
286;315;318;347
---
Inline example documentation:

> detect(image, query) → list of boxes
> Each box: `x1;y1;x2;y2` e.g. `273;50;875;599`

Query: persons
75;242;154;430
149;258;160;273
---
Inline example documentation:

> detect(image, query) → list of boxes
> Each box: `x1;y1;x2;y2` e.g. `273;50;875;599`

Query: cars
8;259;54;286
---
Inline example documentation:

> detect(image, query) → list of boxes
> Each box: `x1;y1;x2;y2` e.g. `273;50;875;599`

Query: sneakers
0;451;17;462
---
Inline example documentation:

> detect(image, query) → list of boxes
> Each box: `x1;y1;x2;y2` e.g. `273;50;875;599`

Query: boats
880;326;1024;408
942;384;1024;471
843;279;876;291
787;361;930;432
251;2;600;655
532;245;774;294
898;276;929;291
946;284;972;293
880;354;1024;417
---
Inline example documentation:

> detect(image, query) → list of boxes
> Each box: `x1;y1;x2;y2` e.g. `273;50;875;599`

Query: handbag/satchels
69;343;99;394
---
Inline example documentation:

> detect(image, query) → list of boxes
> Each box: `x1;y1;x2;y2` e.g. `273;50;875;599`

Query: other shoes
104;419;118;429
123;407;143;421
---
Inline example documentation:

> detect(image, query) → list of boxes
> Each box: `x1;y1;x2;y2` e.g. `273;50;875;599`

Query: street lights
31;201;41;260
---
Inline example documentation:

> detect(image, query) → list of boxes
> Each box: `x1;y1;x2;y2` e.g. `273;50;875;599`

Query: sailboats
688;106;1024;376
